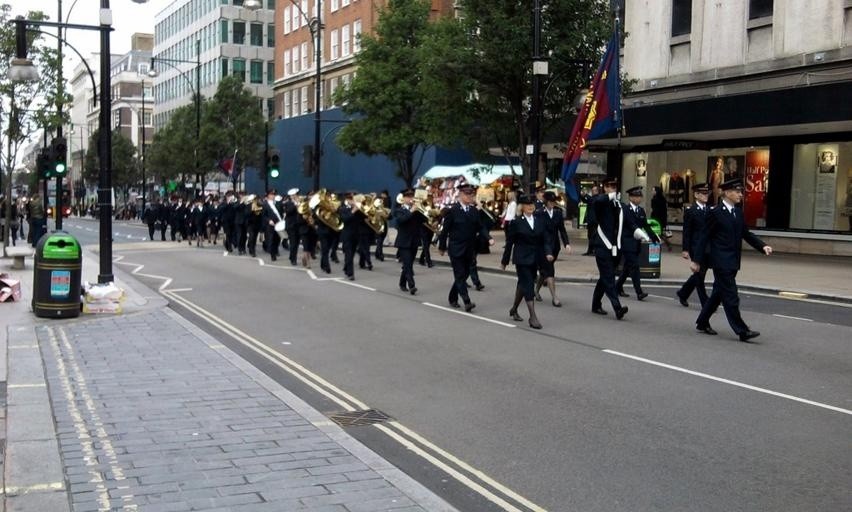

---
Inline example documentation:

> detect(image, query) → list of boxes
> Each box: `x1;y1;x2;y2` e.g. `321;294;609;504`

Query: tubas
298;203;315;226
309;187;344;231
356;194;386;234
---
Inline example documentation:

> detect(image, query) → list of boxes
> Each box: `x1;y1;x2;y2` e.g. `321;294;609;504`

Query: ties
635;207;638;213
731;208;735;218
466;207;469;213
703;206;706;216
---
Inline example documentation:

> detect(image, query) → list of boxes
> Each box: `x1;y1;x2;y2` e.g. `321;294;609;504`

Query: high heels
509;308;524;321
529;317;542;330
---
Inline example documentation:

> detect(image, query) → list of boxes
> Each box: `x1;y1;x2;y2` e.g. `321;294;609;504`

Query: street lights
145;54;201;185
241;0;326;191
67;120;92;136
4;11;115;284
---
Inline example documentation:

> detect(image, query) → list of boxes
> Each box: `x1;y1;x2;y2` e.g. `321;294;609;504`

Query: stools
5;244;34;269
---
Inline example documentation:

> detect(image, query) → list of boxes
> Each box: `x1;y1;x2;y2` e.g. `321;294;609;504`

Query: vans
177;183;196;200
198;181;245;197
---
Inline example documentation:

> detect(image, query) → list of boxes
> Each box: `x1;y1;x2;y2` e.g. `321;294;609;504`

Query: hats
225;187;357;201
456;183;472;194
542;191;558;201
399;187;416;197
690;182;711;194
625;185;645;197
519;194;535;204
717;178;744;192
601;177;619;186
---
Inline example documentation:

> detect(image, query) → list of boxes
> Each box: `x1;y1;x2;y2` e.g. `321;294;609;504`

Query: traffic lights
269;151;280;179
36;153;51;180
255;150;265;169
299;143;314;180
50;135;68;178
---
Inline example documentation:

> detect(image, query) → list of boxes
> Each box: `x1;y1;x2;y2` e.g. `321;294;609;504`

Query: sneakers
249;242;433;295
450;301;460;308
477;284;485;291
465;303;476;312
466;282;472;288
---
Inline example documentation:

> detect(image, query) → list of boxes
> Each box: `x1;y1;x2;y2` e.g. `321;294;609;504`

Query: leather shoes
535;291;543;301
616;305;628;320
591;307;608;315
149;236;218;247
739;330;760;341
618;288;630;297
552;299;562;307
637;292;649;301
696;323;718;336
676;291;689;307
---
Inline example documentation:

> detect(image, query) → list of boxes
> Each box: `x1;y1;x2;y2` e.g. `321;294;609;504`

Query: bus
38;177;72;219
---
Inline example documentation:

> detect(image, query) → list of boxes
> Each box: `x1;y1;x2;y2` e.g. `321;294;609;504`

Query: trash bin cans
615;218;662;279
32;230;82;319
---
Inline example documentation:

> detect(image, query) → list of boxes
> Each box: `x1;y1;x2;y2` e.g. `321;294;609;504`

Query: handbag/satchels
665;231;673;239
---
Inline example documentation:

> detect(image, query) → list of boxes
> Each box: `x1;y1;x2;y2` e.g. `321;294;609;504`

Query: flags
562;21;623;199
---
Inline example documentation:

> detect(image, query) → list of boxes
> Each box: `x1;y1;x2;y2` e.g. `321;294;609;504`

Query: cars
10;180;30;216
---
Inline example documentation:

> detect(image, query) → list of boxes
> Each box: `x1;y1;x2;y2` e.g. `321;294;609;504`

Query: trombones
411;198;440;233
